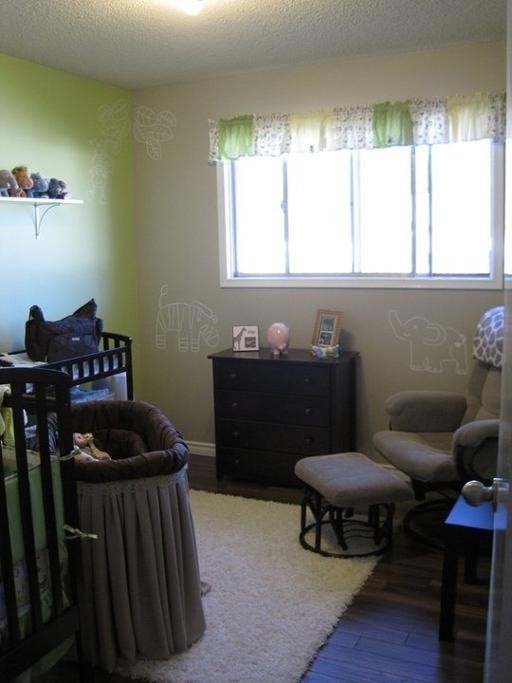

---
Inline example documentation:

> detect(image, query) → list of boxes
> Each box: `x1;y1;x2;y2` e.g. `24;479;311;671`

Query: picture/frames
310;308;345;358
232;325;260;353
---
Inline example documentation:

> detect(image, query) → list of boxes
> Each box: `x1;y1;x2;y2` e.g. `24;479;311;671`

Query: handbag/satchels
24;299;103;363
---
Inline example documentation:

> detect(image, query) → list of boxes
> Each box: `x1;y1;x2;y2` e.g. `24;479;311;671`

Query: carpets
25;486;406;683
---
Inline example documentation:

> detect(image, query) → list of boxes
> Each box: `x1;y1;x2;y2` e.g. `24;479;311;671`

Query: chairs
294;307;504;564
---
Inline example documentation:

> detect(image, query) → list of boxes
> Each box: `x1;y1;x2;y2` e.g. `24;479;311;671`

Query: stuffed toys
1;164;73;200
71;427;113;462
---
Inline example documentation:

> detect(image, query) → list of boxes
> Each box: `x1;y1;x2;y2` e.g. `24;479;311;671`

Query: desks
436;493;494;644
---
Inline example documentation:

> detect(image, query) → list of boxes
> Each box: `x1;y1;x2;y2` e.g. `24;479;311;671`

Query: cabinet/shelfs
205;345;360;502
0;332;133;405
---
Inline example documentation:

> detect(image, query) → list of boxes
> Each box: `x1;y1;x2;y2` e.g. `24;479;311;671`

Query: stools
293;452;413;559
49;397;207;661
